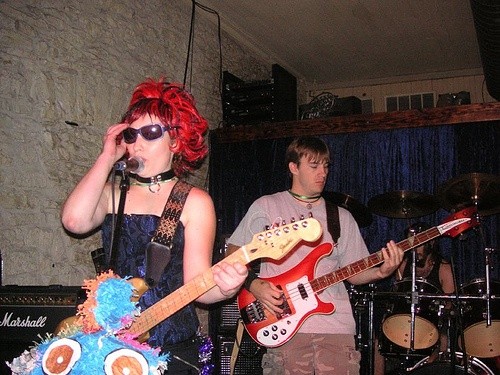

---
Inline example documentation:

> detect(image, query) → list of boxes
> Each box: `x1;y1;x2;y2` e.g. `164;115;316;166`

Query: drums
450;277;500;358
349;291;371;348
405;350;494;375
381;276;447;350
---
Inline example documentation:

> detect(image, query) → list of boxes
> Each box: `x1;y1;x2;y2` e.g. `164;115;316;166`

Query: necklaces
129;168;177;193
288;191;320;209
288;190;321;199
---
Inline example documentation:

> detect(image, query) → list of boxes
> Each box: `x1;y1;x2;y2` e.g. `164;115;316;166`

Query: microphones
114;157;144;174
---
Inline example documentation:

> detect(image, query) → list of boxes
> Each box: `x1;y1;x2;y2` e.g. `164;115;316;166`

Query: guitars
236;204;483;348
52;211;322;344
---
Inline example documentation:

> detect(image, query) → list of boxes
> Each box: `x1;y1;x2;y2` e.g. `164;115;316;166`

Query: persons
226;135;404;375
373;222;455;375
61;74;248;375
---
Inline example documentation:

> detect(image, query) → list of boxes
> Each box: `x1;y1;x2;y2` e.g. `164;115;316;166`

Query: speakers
0;306;80;375
216;333;267;375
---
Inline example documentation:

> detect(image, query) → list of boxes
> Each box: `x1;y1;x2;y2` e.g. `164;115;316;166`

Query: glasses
120;123;182;144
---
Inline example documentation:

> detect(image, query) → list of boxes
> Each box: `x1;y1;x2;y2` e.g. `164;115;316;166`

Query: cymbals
435;172;500;216
321;190;372;228
367;190;439;219
